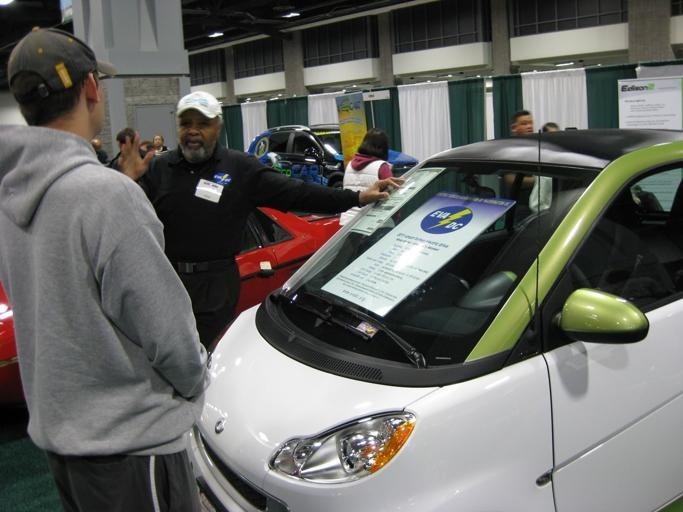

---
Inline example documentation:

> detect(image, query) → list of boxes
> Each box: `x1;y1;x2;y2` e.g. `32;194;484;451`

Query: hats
175;90;222;119
116;128;136;141
6;26;117;103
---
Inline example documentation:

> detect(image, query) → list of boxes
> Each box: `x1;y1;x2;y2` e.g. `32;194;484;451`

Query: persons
337;127;398;248
0;22;206;512
113;88;406;348
528;121;558;214
91;127;170;178
498;109;543;221
